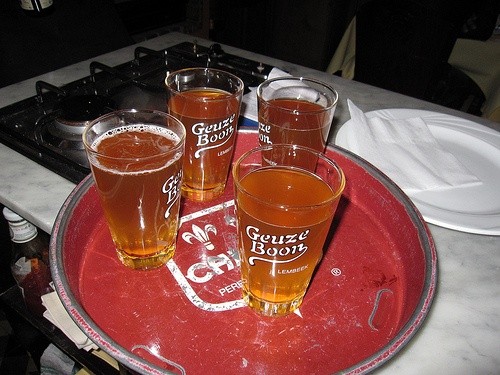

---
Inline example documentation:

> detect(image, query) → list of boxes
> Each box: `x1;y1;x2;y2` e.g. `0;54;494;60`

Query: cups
82;107;187;271
232;143;346;316
164;67;244;202
255;76;338;175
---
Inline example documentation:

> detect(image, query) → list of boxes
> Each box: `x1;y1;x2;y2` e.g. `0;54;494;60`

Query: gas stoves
0;39;274;185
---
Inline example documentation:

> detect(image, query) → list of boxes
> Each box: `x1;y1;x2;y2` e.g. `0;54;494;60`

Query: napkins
240;68;328;123
347;100;482;190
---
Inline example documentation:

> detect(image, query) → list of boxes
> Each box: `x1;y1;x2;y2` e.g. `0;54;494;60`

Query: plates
335;108;500;236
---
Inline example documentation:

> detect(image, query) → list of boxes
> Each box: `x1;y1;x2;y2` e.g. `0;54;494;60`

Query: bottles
3;206;54;318
18;0;55;18
